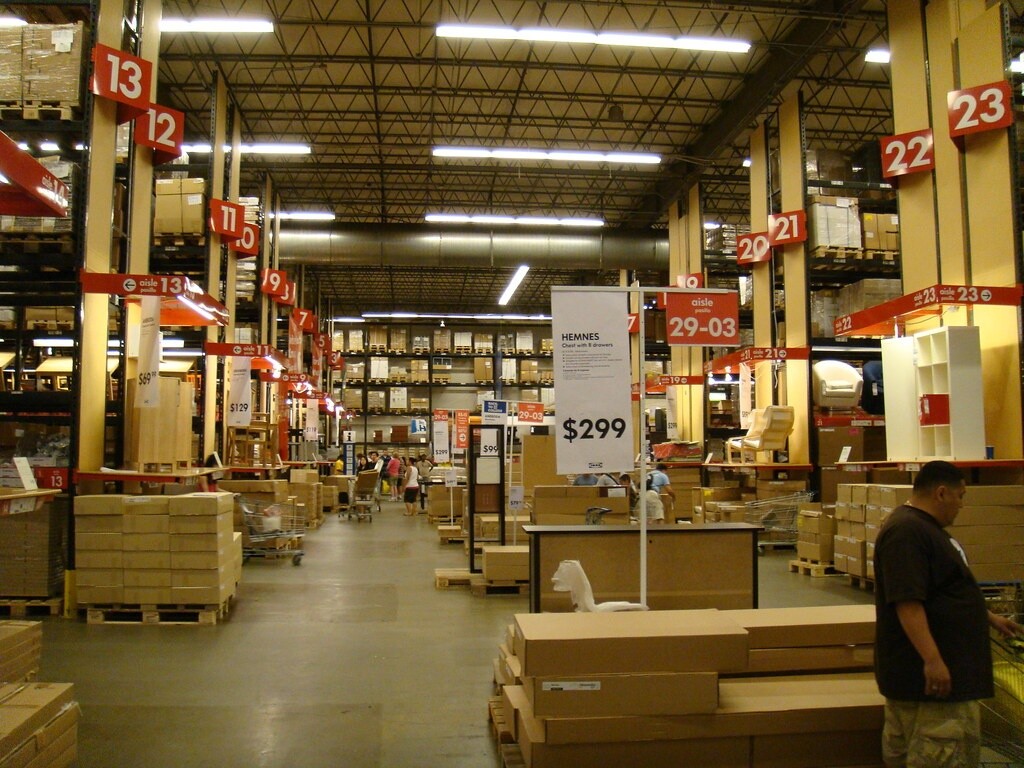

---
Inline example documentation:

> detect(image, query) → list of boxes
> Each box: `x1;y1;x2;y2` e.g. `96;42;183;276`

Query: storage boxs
0;23;1024;768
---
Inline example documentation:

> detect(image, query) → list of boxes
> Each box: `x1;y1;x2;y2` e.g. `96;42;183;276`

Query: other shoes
388;496;402;501
404;512;413;516
376;506;379;510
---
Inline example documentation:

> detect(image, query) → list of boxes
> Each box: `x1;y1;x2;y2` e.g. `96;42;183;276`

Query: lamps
33;13;891;354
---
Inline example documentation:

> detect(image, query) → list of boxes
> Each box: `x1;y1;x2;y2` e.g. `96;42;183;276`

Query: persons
573;474;597;486
334;454;344;475
632;479;664;525
871;460;1024;768
596;473;637;495
647;464;676;502
357;448;434;517
619;474;635;516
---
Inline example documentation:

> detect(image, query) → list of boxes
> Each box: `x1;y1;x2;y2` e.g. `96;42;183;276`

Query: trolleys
747;489;815;555
973;580;1024;767
234;496;311;567
345;488;376;524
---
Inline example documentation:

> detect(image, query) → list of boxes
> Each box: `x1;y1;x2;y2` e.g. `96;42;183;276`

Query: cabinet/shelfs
1;0;1024;613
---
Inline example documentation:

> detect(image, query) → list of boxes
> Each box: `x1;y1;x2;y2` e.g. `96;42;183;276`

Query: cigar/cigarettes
1006;614;1015;620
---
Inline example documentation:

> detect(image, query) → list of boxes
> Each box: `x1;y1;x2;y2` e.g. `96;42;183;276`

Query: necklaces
904;500;912;506
